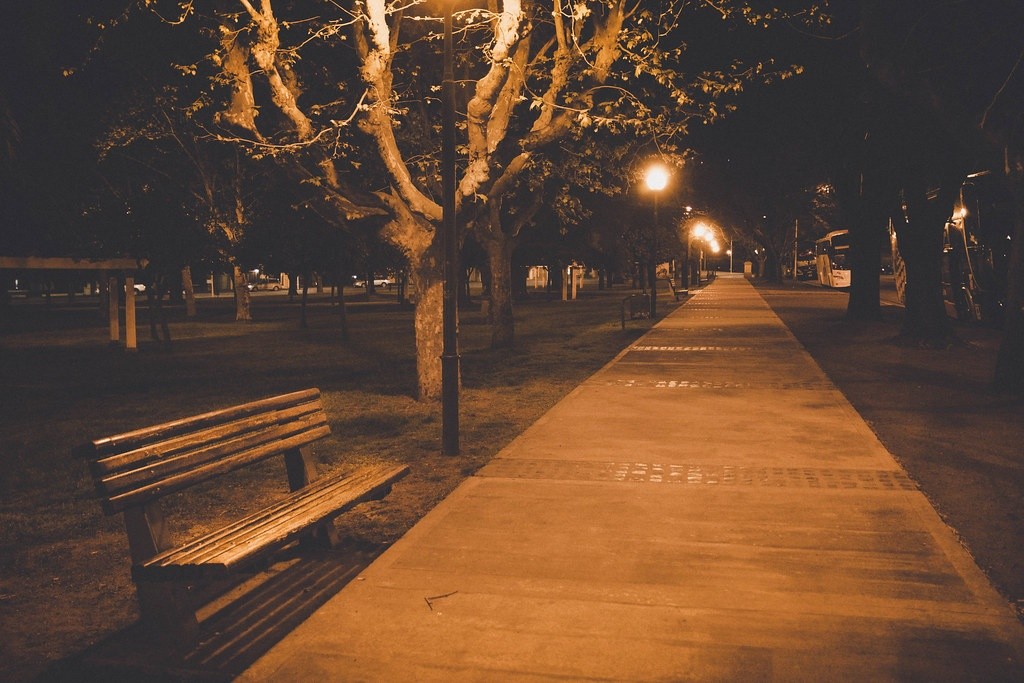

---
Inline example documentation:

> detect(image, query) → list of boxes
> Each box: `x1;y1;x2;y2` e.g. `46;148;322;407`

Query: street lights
684;205;692;295
694;225;705;287
645;166;668;319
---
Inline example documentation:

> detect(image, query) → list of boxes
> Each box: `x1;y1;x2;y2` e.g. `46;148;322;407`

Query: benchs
72;386;413;666
668;279;688;299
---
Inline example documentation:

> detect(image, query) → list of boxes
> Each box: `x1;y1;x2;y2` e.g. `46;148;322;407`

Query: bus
815;229;851;289
889;170;1020;321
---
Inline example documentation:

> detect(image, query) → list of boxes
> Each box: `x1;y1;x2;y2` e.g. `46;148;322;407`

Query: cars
94;283;146;296
353;273;390;289
246;277;283;291
